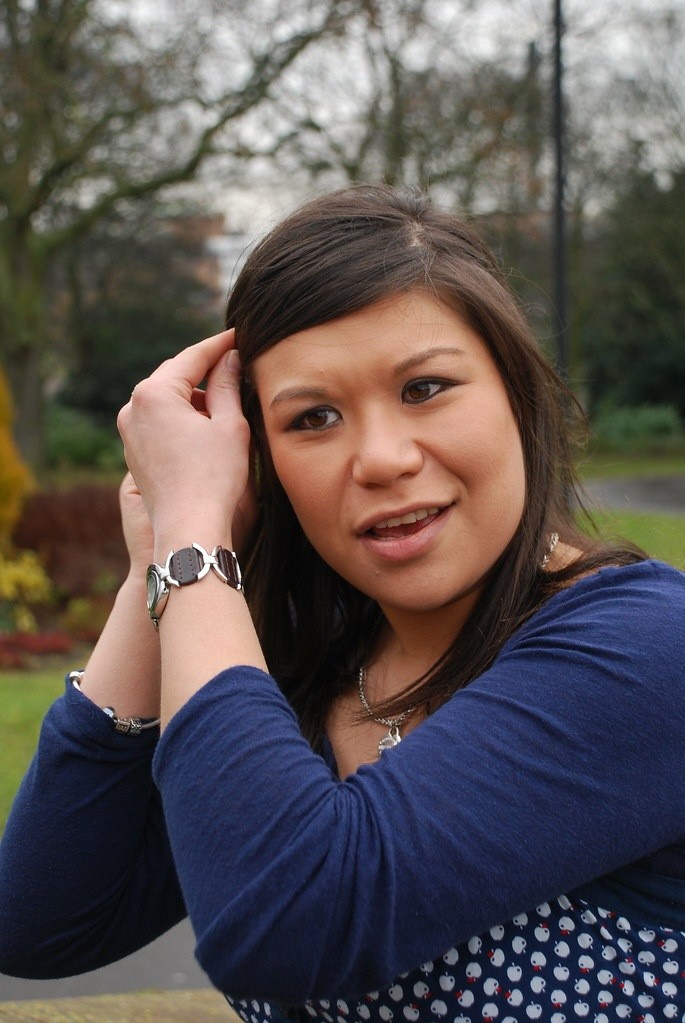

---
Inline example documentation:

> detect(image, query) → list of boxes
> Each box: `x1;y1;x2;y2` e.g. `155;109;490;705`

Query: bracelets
66;666;162;735
143;541;246;631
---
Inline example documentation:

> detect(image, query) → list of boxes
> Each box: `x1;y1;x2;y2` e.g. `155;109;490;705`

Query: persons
0;178;684;1023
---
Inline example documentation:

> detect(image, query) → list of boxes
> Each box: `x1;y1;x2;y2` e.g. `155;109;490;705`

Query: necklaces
356;528;559;755
